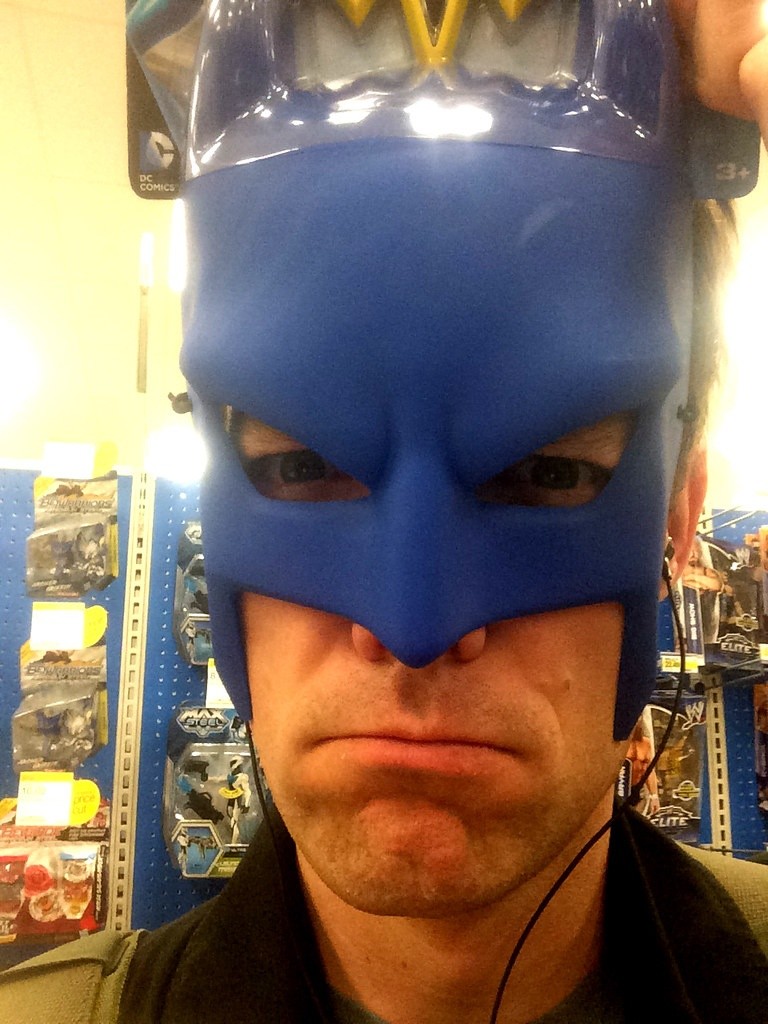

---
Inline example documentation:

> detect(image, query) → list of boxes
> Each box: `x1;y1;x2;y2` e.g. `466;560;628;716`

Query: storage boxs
163;699;266;880
655;539;705;675
615;689;713;847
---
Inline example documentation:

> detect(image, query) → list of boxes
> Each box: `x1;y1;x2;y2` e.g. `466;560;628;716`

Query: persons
0;1;768;1024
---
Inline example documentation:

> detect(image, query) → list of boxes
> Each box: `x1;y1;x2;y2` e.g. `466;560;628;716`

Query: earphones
660;536;676;580
